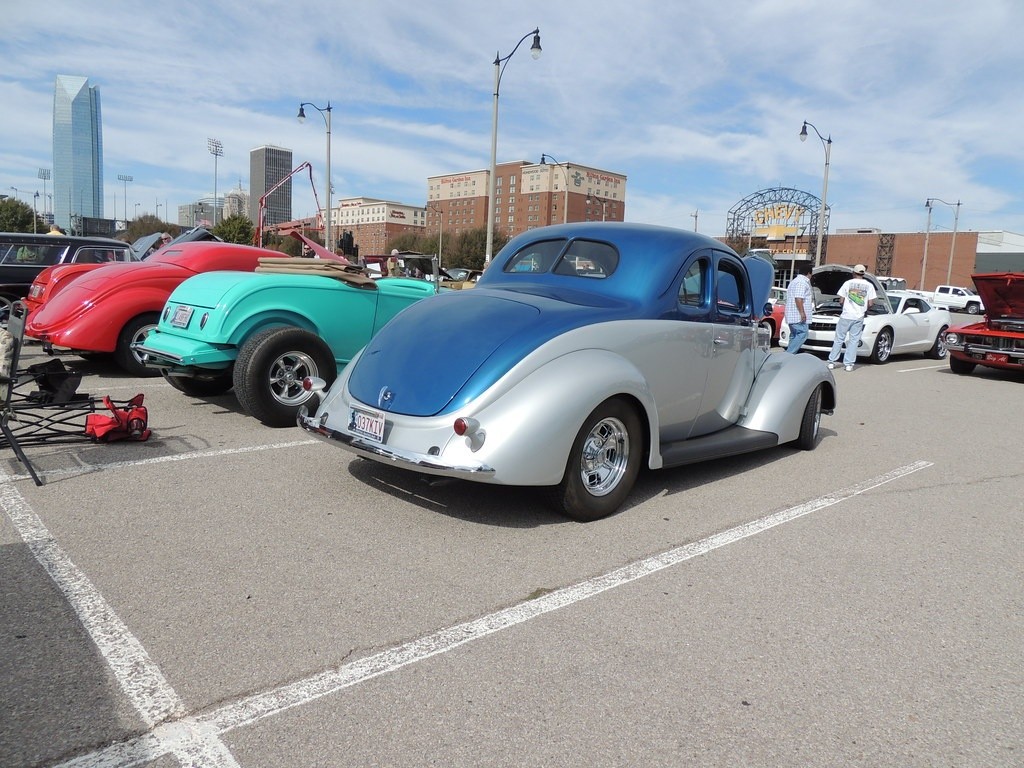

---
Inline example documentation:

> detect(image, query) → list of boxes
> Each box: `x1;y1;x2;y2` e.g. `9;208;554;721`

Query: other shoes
828;362;836;369
846;365;853;372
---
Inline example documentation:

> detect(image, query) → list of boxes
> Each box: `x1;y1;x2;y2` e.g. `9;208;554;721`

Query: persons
406;266;409;277
160;232;173;249
784;264;813;355
46;224;64;235
580;263;593;274
302;244;314;258
827;264;877;372
387;249;400;277
17;245;41;264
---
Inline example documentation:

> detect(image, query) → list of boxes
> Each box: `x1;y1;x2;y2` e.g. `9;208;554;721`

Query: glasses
304;248;309;250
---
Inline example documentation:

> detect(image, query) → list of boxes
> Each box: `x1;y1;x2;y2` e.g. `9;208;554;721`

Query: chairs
0;297;151;487
539;257;579;276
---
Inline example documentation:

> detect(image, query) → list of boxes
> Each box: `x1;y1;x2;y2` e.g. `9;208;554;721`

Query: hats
854;264;866;275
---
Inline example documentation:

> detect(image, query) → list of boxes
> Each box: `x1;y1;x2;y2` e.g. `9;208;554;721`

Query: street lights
586;192;606;222
925;198;963;286
424;204;443;268
208;138;224;227
38;169;51;219
33;194;40;234
482;27;542;276
298;101;330;251
11;186;17;201
135;203;140;221
540;154;571;224
118;175;133;232
799;121;833;266
156;204;162;220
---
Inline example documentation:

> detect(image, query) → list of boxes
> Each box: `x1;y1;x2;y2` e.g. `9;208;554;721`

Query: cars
940;271;1024;374
20;226;351;379
297;222;837;522
778;265;950;365
718;286;786;341
397;251;482;282
131;251;457;428
0;232;162;321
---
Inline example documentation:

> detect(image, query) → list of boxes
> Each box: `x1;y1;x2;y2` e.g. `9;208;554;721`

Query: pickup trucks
906;286;987;314
